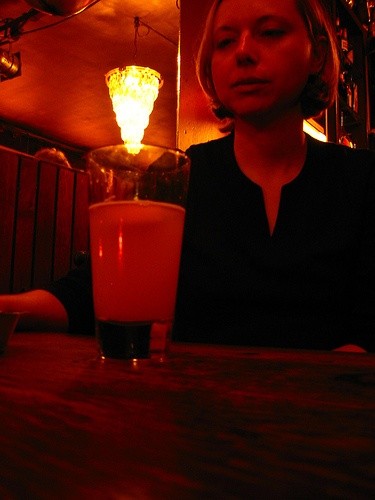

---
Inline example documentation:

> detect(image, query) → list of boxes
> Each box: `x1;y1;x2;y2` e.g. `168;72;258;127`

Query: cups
86;144;190;365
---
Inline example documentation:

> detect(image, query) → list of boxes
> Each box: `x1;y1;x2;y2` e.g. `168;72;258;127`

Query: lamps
103;16;163;155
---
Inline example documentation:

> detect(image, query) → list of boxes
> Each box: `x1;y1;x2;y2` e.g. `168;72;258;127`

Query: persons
0;0;375;354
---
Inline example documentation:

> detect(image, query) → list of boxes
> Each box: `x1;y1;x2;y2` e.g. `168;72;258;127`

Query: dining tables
0;328;375;498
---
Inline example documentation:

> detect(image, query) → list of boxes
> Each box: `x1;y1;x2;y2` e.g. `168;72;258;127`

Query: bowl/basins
0;312;18;356
